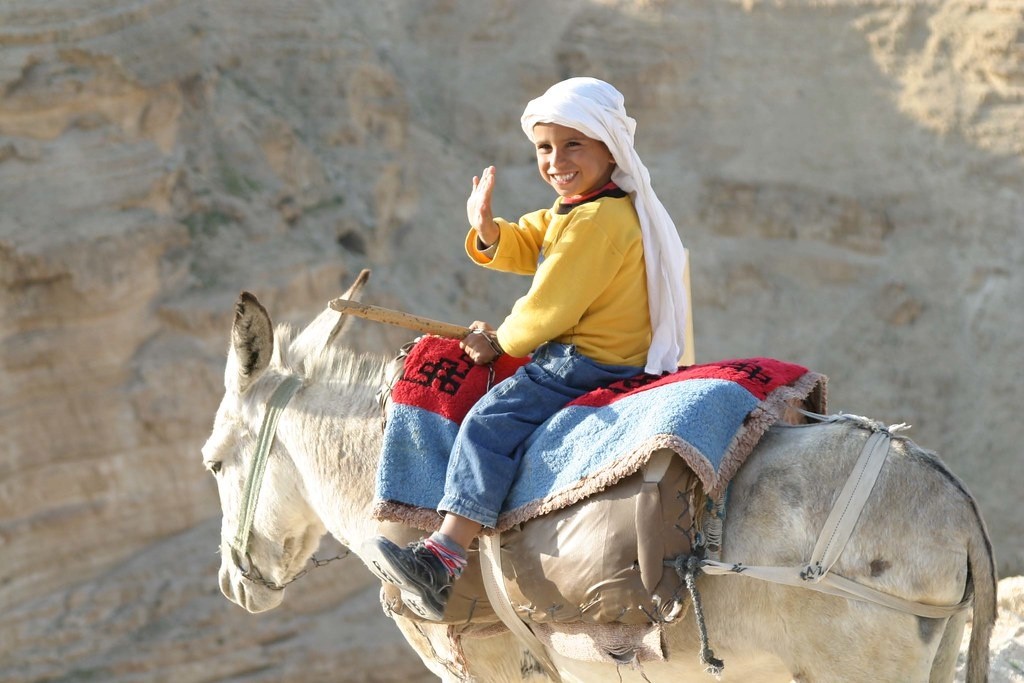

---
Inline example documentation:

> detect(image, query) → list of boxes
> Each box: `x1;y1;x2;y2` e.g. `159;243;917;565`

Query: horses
201;267;1000;683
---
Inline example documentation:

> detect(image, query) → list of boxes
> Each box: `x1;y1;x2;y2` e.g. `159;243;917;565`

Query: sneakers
363;537;455;623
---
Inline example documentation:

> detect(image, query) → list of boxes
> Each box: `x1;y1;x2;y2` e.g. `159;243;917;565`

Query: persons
377;77;695;623
